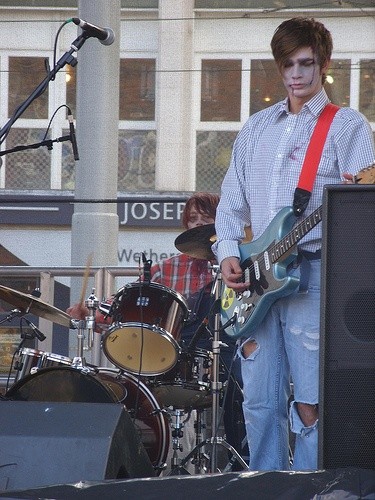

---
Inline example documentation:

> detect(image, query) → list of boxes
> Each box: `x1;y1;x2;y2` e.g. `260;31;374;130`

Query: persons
210;17;375;473
67;192;222;324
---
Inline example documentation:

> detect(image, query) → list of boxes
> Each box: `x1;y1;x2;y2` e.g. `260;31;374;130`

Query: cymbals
174;223;217;261
0;285;75;328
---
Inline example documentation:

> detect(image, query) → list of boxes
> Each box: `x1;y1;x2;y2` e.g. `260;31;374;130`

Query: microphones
141;253;152;280
190;311;211;346
73;17;116;46
67;109;80;161
25;318;47;342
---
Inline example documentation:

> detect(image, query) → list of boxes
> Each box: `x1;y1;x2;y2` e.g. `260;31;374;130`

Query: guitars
220;160;375;338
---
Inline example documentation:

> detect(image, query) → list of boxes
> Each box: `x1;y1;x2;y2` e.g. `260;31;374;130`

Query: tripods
166;268;249;475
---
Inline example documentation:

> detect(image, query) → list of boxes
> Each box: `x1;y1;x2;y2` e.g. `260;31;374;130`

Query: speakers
0;400;156;492
319;184;375;474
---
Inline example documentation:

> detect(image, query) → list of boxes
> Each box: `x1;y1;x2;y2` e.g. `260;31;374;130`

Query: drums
2;365;170;479
14;347;95;382
148;346;226;410
99;280;190;377
